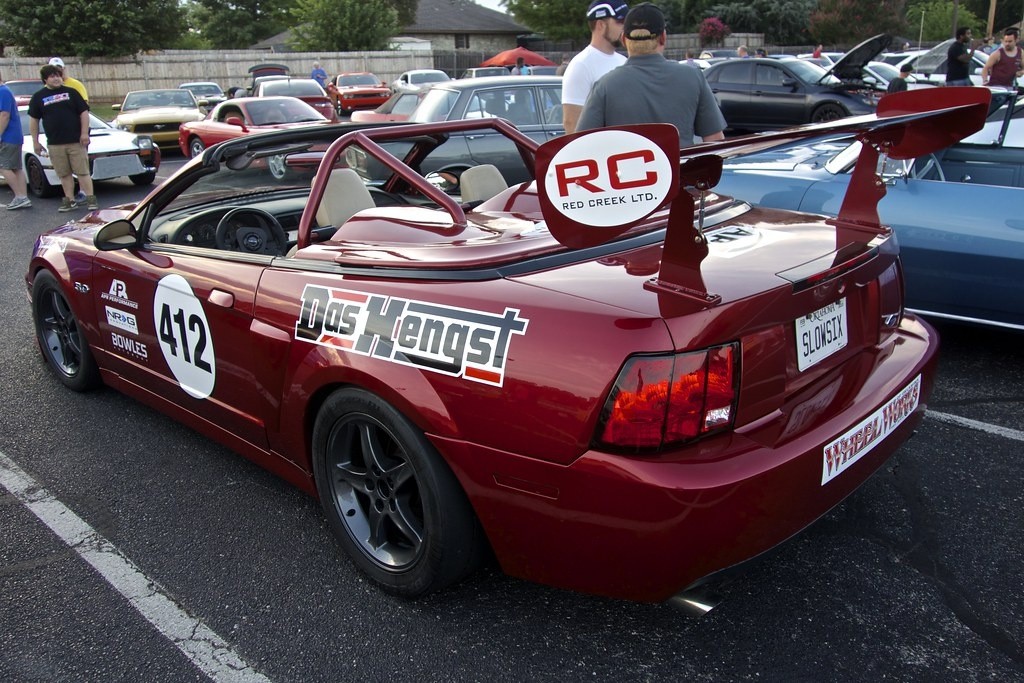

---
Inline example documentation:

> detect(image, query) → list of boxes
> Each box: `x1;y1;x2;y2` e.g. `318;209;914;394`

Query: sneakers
58;197;78;211
5;196;32;210
86;195;99;210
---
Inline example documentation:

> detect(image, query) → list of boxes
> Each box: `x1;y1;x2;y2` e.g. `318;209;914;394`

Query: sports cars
674;88;1024;358
23;85;992;603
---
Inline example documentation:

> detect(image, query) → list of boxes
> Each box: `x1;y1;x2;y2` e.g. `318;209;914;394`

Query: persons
327;78;339;113
27;65;98;212
755;48;767;58
982;28;1024;91
311;62;327;90
738;46;750;58
562;0;629;134
557;54;569;76
813;45;822;67
886;64;913;94
685;51;701;70
0;72;32;210
504;58;526;75
888;37;912;52
979;38;1002;55
946;27;979;85
575;3;727;149
228;87;246;99
44;58;89;204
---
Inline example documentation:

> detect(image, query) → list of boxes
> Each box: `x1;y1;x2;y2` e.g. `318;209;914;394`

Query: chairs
285;168;377;259
459;164;509;210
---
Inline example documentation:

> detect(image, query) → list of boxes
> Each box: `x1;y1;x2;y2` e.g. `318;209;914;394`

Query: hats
49;57;65;69
623;3;666;39
586;0;637;22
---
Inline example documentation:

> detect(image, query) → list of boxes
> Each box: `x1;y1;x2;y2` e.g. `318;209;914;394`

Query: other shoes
75;192;87;205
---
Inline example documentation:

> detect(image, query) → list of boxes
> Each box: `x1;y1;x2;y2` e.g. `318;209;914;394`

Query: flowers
699;18;732;48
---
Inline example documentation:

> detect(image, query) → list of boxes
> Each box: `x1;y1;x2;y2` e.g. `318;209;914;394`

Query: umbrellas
481;46;558;67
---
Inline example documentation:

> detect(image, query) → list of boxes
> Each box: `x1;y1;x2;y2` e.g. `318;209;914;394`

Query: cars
675;38;1024;93
0;105;161;199
700;32;893;134
4;79;44;107
178;81;228;112
105;88;210;149
344;74;566;197
234;62;560;124
178;96;349;175
